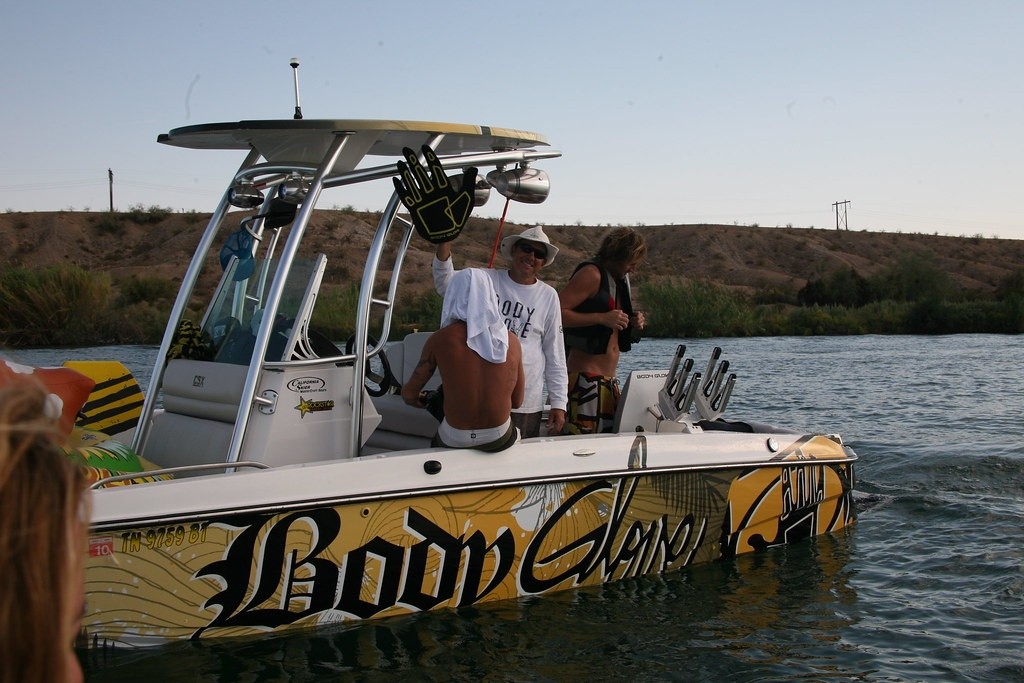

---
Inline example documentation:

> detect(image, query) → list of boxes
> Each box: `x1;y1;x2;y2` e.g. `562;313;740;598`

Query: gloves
392;143;478;244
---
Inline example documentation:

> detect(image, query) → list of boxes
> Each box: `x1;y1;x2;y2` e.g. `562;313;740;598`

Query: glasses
516;240;548;260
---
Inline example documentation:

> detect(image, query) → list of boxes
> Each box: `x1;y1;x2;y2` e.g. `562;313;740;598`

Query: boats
1;56;859;674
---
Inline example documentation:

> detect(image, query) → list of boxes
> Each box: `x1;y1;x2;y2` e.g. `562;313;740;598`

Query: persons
0;373;93;683
548;225;644;435
393;226;569;440
401;268;526;452
212;309;318;366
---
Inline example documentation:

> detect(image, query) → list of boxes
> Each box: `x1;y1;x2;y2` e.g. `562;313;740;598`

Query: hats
500;225;559;268
